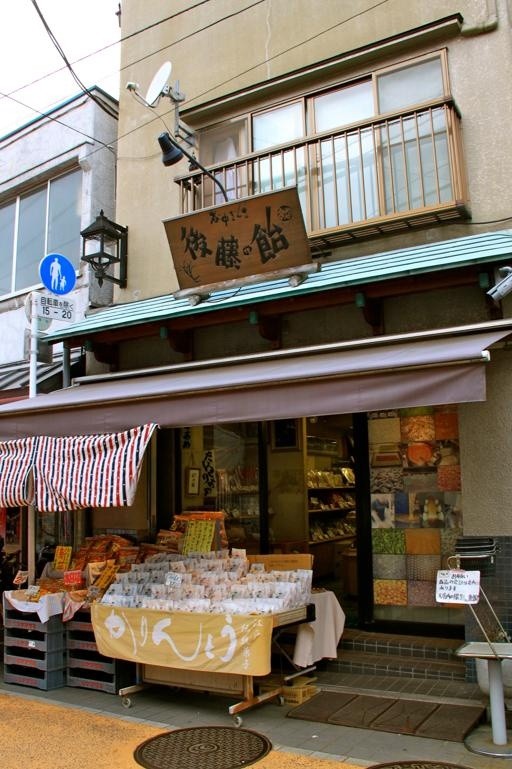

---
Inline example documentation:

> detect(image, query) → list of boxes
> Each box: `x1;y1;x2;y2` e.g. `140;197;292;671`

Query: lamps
155;130;227;204
79;208;128;289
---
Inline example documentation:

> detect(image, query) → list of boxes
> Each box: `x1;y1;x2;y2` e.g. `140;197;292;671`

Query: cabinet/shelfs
307;484;356;588
216;490;259;522
